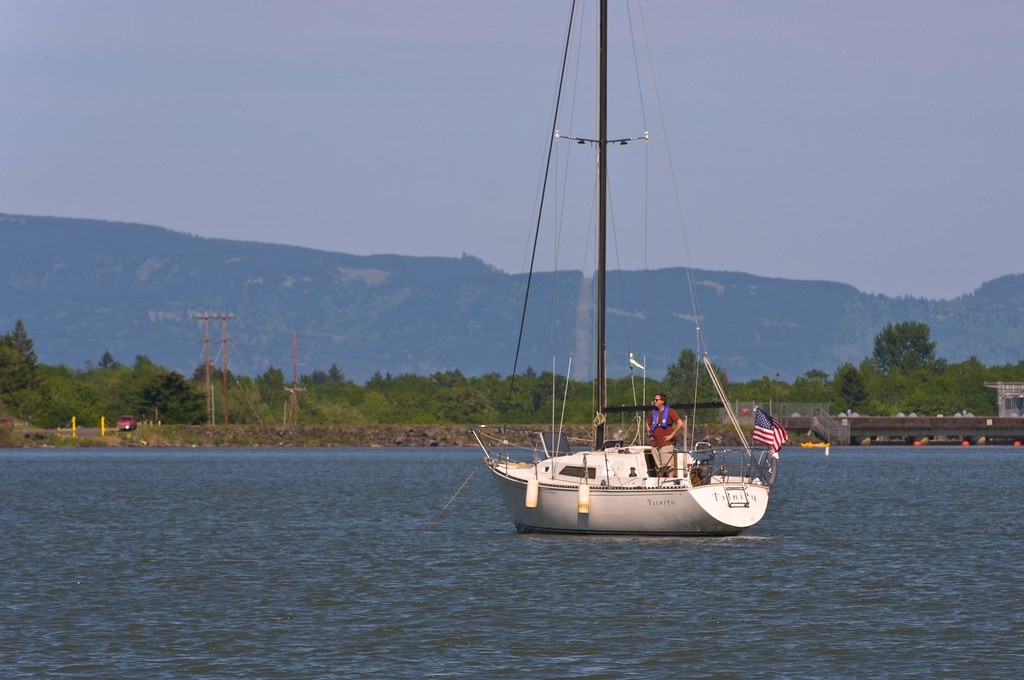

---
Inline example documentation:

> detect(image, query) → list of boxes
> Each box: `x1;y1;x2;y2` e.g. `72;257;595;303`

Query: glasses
654;399;662;402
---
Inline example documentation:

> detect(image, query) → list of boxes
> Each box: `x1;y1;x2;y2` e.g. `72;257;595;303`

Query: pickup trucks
116;415;137;431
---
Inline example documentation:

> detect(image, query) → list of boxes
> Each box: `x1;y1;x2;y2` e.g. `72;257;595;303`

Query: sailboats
470;0;777;536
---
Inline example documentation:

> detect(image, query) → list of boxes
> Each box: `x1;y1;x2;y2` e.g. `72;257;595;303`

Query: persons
645;392;683;478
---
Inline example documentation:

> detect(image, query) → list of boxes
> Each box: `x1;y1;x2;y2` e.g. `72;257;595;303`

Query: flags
752;408;788;452
629;353;645;370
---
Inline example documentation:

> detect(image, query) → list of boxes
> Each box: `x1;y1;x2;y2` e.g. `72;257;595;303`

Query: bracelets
648;434;653;438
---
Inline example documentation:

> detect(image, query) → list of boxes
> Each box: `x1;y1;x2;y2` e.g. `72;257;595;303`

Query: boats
800;442;830;448
